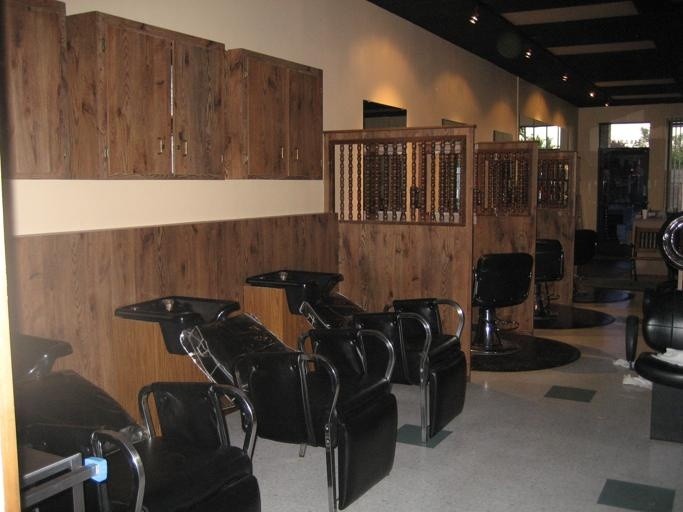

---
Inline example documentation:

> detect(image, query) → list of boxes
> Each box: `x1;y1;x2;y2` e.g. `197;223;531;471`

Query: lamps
467;10;569;84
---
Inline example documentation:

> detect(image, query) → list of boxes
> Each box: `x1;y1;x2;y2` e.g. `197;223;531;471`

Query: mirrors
441;117;473;211
493;131;512;198
514;113;569;210
362;99;406;212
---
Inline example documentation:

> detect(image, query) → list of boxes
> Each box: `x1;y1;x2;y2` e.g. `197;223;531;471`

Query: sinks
246;269;341;314
10;336;72;389
114;296;240;355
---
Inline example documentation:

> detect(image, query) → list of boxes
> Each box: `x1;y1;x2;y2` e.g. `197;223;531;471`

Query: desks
18;443;100;512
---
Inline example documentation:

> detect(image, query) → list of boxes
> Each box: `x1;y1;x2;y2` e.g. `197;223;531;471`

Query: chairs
470;209;668;372
243;268;467;444
624;211;683;390
4;332;261;512
115;296;398;512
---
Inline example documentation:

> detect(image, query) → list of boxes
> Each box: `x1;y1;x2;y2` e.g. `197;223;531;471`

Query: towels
648;346;683;367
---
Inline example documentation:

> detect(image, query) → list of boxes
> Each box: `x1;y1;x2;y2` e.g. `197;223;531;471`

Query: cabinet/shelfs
224;46;323;180
66;10;224;180
0;0;66;178
